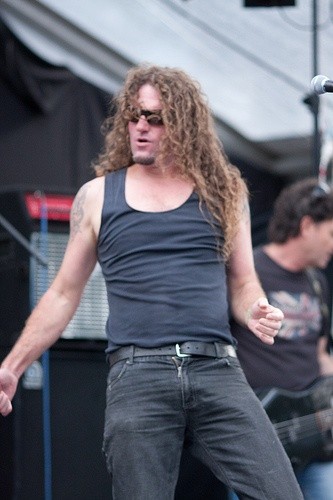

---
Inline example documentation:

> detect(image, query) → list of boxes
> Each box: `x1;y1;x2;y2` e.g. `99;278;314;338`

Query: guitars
254;374;332;473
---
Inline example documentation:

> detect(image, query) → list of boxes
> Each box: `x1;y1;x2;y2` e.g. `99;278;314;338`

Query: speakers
0;190;111;347
0;346;228;500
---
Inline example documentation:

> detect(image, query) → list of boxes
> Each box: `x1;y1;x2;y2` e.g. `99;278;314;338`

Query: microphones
312;75;333;94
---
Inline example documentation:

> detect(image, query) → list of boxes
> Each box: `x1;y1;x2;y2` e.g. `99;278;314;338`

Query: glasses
309;182;331;199
130;110;164;125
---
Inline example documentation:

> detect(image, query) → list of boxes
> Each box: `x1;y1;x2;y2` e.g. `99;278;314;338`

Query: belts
109;341;237;368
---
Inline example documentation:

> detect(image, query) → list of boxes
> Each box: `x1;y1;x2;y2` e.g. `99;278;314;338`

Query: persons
1;66;303;500
225;177;333;500
